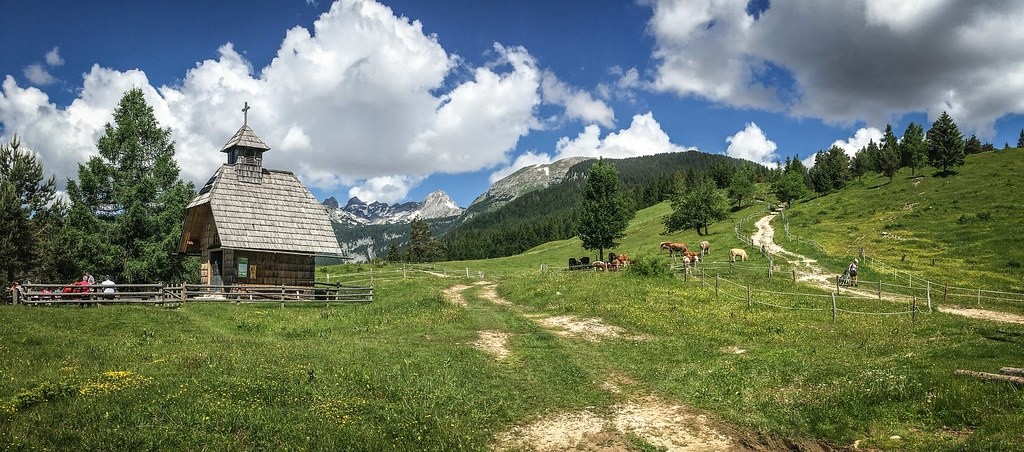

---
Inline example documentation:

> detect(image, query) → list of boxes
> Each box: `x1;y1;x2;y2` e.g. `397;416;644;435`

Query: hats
855;259;858;263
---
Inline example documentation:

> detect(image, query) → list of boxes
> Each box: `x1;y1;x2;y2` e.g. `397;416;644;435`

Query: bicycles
838;269;852;287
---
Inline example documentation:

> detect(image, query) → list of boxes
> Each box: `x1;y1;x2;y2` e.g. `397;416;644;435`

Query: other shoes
851;284;853;286
855;284;858;286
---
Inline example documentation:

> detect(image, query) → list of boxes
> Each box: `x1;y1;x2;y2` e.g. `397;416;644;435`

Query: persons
848;259;858;286
41;281;92;309
83;272;94;285
101;275;117;300
9;280;26;299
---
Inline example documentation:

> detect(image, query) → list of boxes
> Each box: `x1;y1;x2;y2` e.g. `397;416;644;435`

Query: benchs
246;288;304;300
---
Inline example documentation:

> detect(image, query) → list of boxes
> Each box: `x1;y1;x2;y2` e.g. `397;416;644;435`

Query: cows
660;240;711;267
569;253;636;272
729;249;749;262
